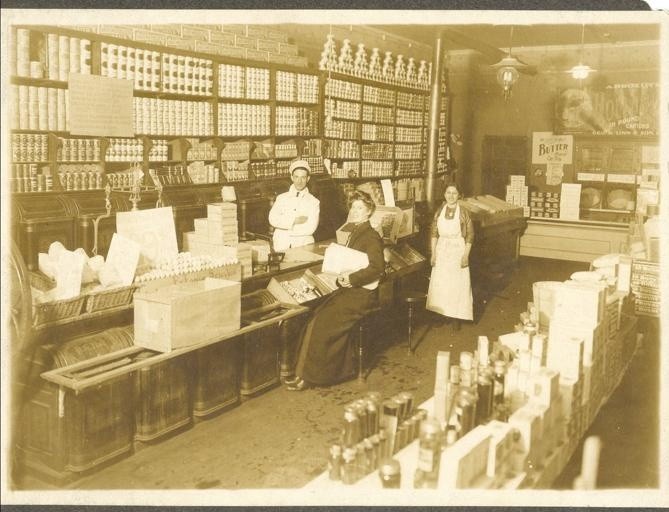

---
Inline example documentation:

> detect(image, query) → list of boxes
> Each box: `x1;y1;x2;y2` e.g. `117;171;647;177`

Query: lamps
560;26;599;81
485;26;537;103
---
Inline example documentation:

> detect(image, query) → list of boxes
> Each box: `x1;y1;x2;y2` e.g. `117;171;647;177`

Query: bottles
250;160;277;177
277;160;293;174
275;144;297;157
105;139;169;161
328;392;429;485
414;360;505;488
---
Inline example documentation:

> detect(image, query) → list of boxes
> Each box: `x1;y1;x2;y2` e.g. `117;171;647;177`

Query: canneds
329;392;427;488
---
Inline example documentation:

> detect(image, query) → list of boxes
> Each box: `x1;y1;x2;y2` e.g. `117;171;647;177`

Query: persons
283;190;384;392
269;160;319;251
430;181;474;332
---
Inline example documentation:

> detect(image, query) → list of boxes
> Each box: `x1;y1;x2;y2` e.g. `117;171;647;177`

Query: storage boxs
68;71;136;139
132;277;241;352
505;174;530;217
643;226;662;263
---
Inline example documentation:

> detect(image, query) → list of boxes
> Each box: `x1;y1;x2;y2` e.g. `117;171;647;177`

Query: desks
519;216;630;263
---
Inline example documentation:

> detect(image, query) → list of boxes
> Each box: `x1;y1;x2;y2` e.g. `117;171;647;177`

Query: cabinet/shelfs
303;268;643;488
11;226;432;489
323;71;452;258
458;192;528;278
10;23;333;271
574;138;642;223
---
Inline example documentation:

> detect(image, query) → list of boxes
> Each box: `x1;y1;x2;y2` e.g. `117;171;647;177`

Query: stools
355;307;388;383
398;289;428;356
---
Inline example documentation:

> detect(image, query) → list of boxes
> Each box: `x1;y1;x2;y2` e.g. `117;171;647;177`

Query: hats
289;160;311;175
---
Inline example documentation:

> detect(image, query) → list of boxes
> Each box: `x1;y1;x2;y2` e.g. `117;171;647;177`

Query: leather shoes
285;377;315;391
